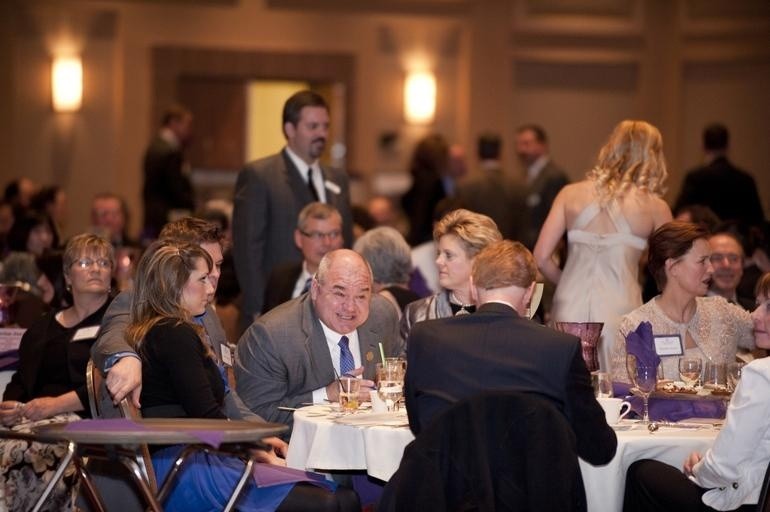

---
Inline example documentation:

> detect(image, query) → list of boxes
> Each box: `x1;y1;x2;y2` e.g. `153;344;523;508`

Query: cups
704;358;726;388
337;377;361;415
590;372;615;400
726;362;744;392
595;398;632;425
556;320;605;372
368;389;389;415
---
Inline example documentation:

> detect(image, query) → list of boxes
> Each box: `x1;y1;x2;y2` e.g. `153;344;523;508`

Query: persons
141;103;196;245
532;119;673;370
673;125;770;258
398;121;570;267
1;178;770;512
232;92;352;333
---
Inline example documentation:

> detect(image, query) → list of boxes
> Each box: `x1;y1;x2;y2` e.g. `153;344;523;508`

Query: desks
30;417;290;512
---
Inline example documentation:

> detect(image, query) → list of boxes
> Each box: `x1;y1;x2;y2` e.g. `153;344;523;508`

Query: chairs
123;393;158;495
735;462;770;512
60;358;121;416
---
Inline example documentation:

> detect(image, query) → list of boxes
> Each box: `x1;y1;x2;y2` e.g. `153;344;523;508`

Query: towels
251;455;327;490
620;321;661;390
624;393;727;421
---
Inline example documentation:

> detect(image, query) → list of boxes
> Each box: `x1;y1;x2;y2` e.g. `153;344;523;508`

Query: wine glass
384;357;407;418
678;356;703;387
375;362;405;421
625;353;663;427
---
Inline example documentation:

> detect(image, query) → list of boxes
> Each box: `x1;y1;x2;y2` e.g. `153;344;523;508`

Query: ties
308;168;319;201
337;336;355;375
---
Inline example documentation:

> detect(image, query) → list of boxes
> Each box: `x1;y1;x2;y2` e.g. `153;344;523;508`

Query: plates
606;420;633;431
628;384;713;402
328;400;372;412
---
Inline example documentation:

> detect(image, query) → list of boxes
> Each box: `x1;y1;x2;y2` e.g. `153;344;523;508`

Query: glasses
69;256;111;269
